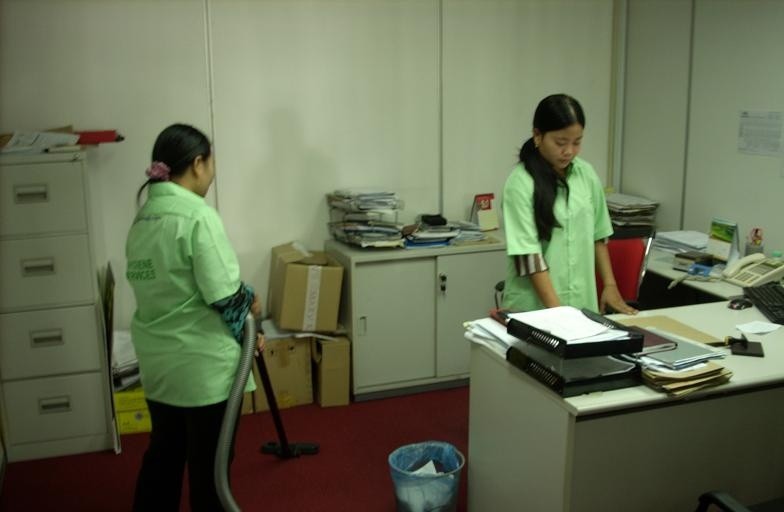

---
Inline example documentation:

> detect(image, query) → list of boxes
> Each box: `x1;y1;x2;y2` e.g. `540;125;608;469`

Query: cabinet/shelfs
324;238;510;402
1;146;121;464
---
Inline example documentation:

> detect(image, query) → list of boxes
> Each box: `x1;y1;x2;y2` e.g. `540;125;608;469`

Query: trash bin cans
388;442;465;512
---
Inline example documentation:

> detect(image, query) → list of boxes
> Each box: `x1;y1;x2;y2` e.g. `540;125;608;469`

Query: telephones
721;253;784;288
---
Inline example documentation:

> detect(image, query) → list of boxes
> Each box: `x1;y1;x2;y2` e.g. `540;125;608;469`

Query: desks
467;242;784;512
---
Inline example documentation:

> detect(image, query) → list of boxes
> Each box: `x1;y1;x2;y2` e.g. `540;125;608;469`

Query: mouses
728;298;753;310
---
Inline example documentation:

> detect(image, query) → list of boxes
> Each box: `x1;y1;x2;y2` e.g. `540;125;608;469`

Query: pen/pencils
44;146;82;153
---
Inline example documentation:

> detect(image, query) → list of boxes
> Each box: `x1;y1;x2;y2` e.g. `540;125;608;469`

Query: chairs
597;230;659;311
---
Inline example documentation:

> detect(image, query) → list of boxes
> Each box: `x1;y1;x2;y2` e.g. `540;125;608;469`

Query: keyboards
743;285;784;324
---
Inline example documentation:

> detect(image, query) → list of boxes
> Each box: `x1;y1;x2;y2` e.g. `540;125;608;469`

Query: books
462;306;734;399
327;190;500;249
652;230;709;254
606;194;660;226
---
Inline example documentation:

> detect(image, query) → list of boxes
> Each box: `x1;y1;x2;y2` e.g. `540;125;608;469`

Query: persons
126;124;265;512
501;94;639;315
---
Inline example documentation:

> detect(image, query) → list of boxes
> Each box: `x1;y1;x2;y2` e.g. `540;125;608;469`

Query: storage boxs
268;237;349;332
112;319;349;437
